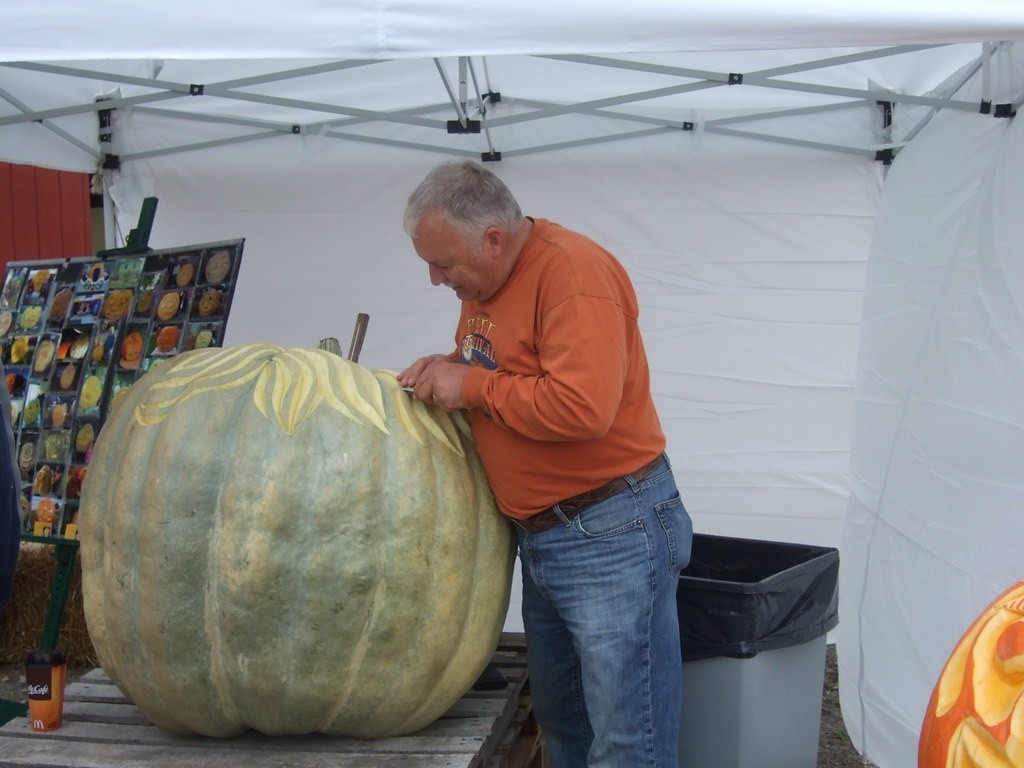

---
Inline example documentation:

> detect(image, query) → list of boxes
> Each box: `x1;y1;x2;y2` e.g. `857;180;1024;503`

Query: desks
0;631;526;768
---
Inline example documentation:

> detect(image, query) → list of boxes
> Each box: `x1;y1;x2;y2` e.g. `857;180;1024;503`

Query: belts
518;453;665;534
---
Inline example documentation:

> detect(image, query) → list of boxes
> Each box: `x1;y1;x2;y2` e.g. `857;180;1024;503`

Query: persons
103;326;116;362
395;160;693;768
13;368;25;395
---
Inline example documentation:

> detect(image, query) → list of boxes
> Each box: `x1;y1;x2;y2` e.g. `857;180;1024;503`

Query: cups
24;648;67;732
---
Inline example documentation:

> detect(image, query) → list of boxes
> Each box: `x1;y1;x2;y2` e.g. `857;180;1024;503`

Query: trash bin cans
679;533;840;768
